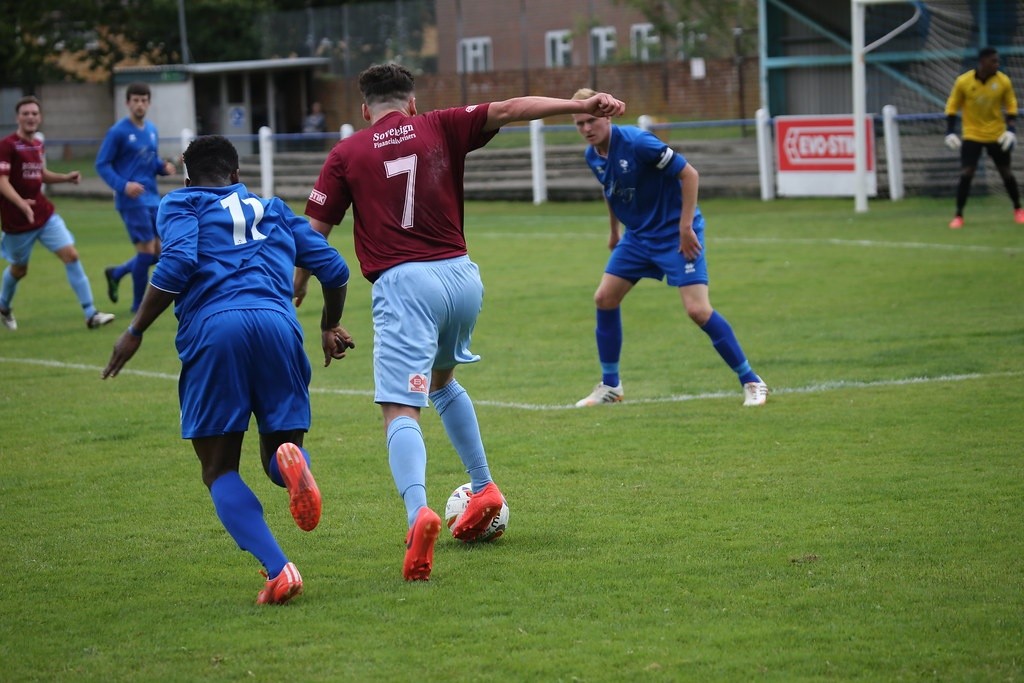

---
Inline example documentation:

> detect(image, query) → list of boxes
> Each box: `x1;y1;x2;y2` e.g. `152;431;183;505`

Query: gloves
997;128;1018;152
944;134;961;150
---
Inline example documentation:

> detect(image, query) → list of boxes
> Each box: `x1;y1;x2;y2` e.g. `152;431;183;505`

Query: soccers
443;481;510;544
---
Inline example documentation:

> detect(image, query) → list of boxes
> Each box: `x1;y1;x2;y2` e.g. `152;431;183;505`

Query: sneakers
86;311;115;329
451;482;504;543
950;215;965;230
744;374;770;407
276;443;322;533
255;561;303;605
577;380;626;408
104;267;120;303
0;305;18;331
1013;207;1024;223
403;504;442;582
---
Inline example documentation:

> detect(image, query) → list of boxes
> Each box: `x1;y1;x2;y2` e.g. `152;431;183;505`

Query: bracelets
129;325;144;336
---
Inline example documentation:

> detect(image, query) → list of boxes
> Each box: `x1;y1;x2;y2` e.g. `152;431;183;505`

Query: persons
95;83;176;314
570;88;768;409
101;135;355;605
0;100;115;330
292;63;625;581
302;100;325;151
945;47;1024;229
